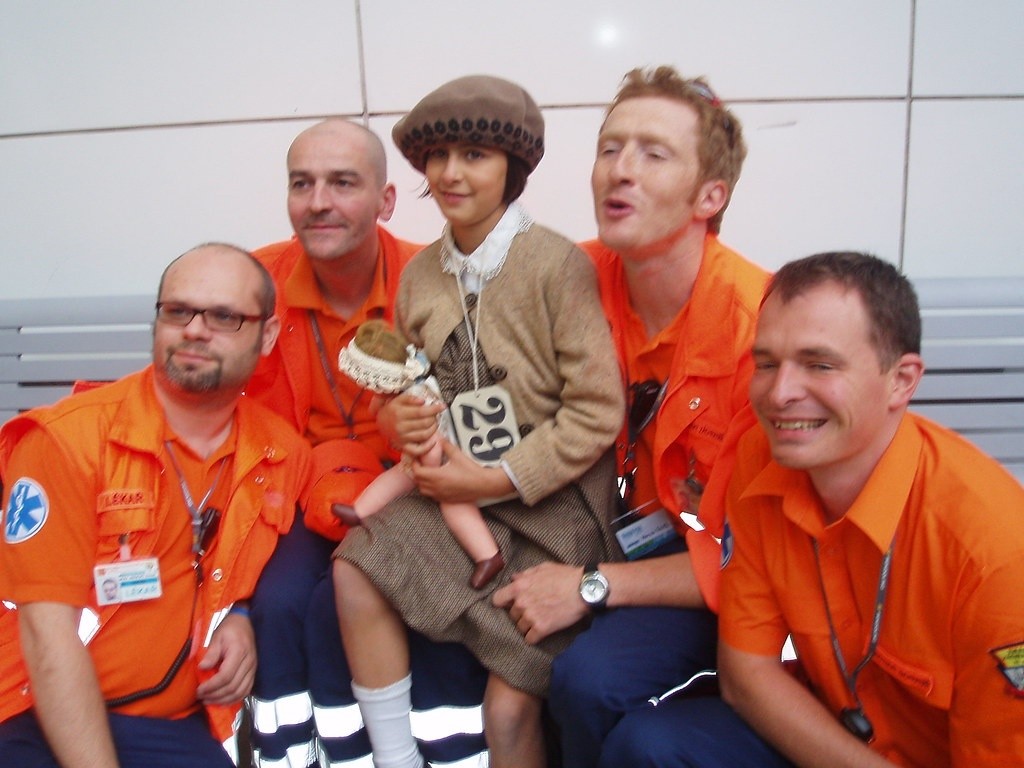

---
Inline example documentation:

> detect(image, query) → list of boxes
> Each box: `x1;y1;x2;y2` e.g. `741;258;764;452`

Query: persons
330;319;505;589
600;251;1024;768
330;75;626;768
403;65;773;768
0;242;312;768
243;119;434;768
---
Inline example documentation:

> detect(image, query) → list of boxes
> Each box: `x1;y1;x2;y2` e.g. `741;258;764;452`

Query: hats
392;75;544;173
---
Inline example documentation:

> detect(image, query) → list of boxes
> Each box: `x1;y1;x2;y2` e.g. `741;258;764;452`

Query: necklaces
308;308;381;438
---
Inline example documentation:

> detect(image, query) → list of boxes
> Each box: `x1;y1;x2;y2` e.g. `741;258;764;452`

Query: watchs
578;562;609;615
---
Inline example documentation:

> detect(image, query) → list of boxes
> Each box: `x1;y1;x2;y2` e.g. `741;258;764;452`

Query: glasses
156;302;265;333
